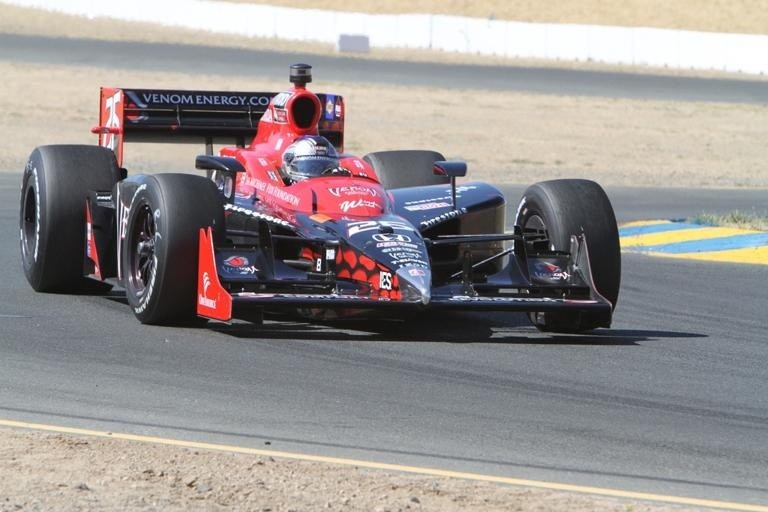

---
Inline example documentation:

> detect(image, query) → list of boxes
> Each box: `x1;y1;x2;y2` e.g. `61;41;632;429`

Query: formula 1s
18;61;623;337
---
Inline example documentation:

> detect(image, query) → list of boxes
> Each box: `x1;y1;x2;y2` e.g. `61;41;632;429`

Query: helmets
282;135;338;181
